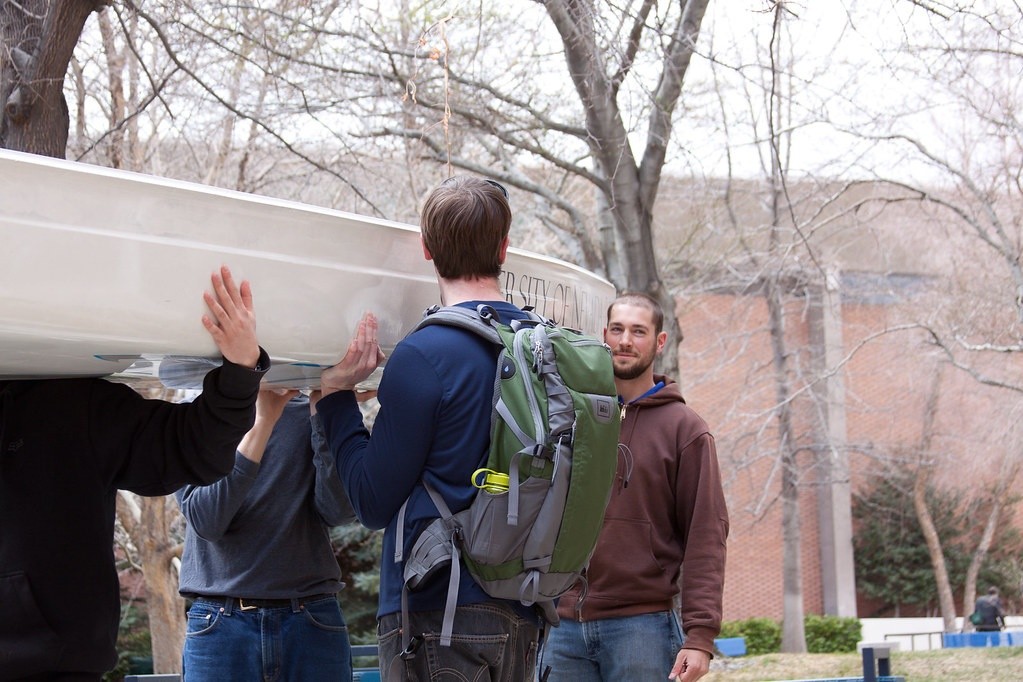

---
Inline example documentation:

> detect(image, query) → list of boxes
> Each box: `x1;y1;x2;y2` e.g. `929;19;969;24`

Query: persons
314;176;559;682
535;293;730;682
175;390;377;682
0;265;271;682
975;586;1006;631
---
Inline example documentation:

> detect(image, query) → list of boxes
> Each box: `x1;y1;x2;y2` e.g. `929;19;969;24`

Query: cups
471;468;510;495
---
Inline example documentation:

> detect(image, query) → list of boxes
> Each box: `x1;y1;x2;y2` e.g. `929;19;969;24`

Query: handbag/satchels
969;607;982;625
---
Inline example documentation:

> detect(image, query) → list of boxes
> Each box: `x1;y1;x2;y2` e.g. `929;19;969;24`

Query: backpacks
391;301;636;658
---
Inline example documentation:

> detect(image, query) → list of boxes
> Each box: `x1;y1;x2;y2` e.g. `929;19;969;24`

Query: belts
195;594;336;611
